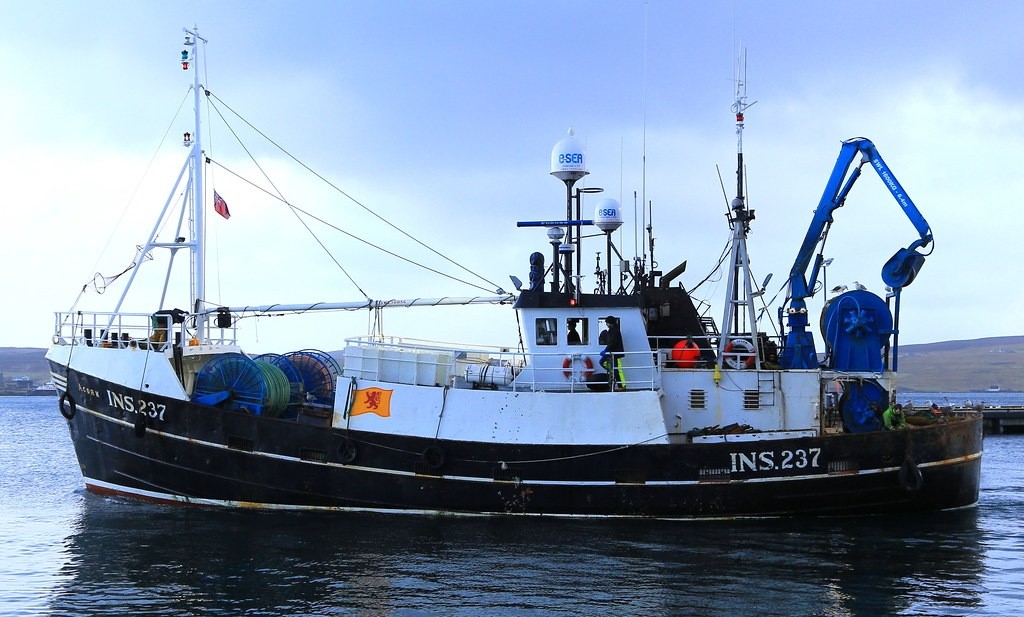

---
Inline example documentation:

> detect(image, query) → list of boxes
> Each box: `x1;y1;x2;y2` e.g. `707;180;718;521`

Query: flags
214;189;231;220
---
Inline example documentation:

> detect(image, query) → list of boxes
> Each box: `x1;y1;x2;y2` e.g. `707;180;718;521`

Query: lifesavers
337;439;359;464
898;463;924;494
422;445;447;471
724;339;755;369
562;353;592;385
58;392;75;420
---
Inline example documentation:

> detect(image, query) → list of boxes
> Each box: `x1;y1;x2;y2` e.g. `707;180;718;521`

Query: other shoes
614;387;625;391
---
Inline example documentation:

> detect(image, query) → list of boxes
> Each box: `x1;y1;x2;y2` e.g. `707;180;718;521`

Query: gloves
600;350;606;356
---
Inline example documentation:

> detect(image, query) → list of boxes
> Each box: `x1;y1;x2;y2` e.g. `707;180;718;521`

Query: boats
44;24;984;531
986;384;1001;393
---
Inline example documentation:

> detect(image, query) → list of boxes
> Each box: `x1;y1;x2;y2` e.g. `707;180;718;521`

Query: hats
605;316;616;324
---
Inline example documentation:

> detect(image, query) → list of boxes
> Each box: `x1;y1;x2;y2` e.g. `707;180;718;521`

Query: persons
146;308;189;350
567;320;581;345
599;330;607;344
599;316;626;391
871;402;880;414
882;404;905;431
932;403;940;413
